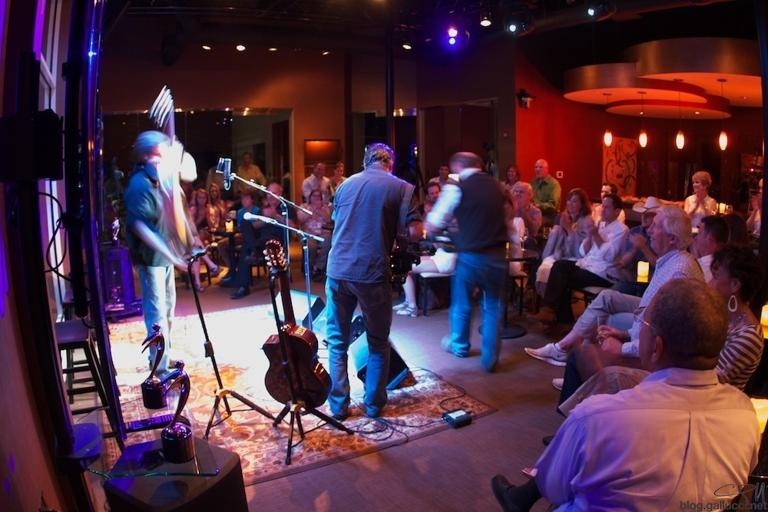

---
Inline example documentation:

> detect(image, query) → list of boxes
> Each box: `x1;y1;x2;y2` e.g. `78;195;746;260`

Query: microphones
223;158;232;190
201;245;211;254
243;211;272;224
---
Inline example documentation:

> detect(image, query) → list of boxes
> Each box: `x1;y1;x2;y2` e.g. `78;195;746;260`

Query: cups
543;227;551;239
726;205;733;214
598;314;611;345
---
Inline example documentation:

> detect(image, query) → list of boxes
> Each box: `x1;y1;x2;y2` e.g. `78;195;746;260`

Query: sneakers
524;341;567;367
551;378;564;391
441;334;464;358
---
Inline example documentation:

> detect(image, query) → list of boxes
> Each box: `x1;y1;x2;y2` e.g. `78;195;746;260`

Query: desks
208;227;244;288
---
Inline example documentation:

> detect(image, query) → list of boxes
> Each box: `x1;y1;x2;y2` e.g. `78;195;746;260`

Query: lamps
602;75;728;152
393;0;606;51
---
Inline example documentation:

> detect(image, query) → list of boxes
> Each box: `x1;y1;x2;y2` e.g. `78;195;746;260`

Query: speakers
348;330;409;390
102;245;136;307
311;304;365;347
267;289;326;328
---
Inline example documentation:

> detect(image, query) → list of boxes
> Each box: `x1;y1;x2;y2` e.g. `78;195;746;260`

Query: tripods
270;270;355;465
187;256;282;441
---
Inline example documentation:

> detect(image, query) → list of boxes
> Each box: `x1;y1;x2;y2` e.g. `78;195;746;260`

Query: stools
54;319;110;415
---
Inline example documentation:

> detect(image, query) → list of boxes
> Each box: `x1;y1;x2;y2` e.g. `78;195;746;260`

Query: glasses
632;306;652;331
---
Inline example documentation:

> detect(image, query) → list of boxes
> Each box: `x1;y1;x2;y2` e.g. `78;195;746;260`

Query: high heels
396;307;418;318
392;302;408;311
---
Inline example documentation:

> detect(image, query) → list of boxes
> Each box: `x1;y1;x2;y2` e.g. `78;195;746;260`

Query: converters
443;409;473;428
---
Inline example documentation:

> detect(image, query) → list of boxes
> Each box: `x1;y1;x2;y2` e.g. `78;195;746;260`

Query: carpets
57;303;499;486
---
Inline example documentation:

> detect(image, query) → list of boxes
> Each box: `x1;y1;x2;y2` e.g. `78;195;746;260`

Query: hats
632;195;663;214
133;130;167;159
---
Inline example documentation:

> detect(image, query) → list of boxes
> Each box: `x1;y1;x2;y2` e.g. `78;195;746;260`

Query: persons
393;158;768;446
297;189;331;282
325;142;424;420
105;152;289;299
124;131;195;386
302;163;330;200
491;279;762;512
328;161;348;191
424;152;520;373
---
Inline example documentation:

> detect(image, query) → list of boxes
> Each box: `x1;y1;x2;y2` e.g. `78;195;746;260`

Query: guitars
262;239;332;409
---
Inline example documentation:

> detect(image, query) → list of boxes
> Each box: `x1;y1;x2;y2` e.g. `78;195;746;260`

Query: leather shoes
542;435;553;447
230;284;251;299
491;474;523;511
220;269;236;285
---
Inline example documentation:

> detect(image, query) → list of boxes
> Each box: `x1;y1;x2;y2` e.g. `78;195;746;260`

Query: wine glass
519;228;528;251
225;213;232;232
